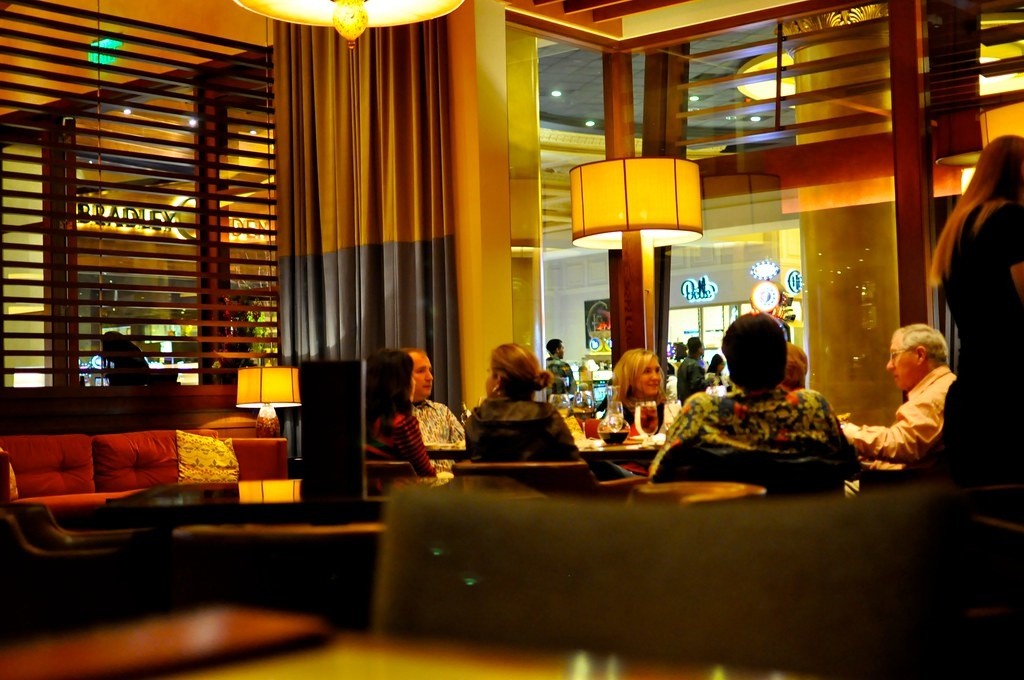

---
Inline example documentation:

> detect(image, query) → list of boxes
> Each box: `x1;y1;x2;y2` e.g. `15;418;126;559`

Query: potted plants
774;3;893;145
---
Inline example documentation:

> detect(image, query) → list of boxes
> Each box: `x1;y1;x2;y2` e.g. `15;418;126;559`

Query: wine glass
572;391;594;439
635;401;659;449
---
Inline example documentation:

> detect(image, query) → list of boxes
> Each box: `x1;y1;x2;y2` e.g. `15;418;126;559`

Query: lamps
568;155;704;356
230;0;465;50
235;360;302;438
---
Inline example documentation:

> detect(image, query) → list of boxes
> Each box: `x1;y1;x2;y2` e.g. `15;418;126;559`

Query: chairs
651;435;845;502
368;483;967;680
941;482;1024;680
364;461;420;495
451;458;649;502
0;500;157;639
168;518;382;631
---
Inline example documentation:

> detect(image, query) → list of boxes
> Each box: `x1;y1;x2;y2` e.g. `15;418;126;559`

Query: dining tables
425;433;666;463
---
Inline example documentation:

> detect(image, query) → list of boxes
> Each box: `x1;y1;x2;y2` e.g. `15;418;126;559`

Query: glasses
889;350;907;361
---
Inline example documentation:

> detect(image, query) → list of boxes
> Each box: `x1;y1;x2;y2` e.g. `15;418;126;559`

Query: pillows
176;429;240;484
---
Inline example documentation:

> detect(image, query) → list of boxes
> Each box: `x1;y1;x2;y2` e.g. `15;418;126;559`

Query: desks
103;482;380;529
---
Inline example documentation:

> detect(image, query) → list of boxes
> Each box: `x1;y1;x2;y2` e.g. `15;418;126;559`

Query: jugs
597;386;630;445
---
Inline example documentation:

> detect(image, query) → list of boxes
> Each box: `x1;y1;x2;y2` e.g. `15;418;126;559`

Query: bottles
460;402;471;424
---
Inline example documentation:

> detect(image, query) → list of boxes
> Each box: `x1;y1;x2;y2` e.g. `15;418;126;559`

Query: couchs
0;429;289;530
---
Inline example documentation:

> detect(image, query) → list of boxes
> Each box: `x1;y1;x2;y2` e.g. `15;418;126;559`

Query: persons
167;328;176;336
465;344;580;463
218;349;243;384
649;314;862;483
942;134;1024;484
842;324;958;463
265;359;272;368
365;350;437;498
400;347;466;488
592;348;682;445
100;332;148;387
546;339;577;401
666;337;808;408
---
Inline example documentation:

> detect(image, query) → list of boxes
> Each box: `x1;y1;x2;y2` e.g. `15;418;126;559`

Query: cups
549;394;571;419
